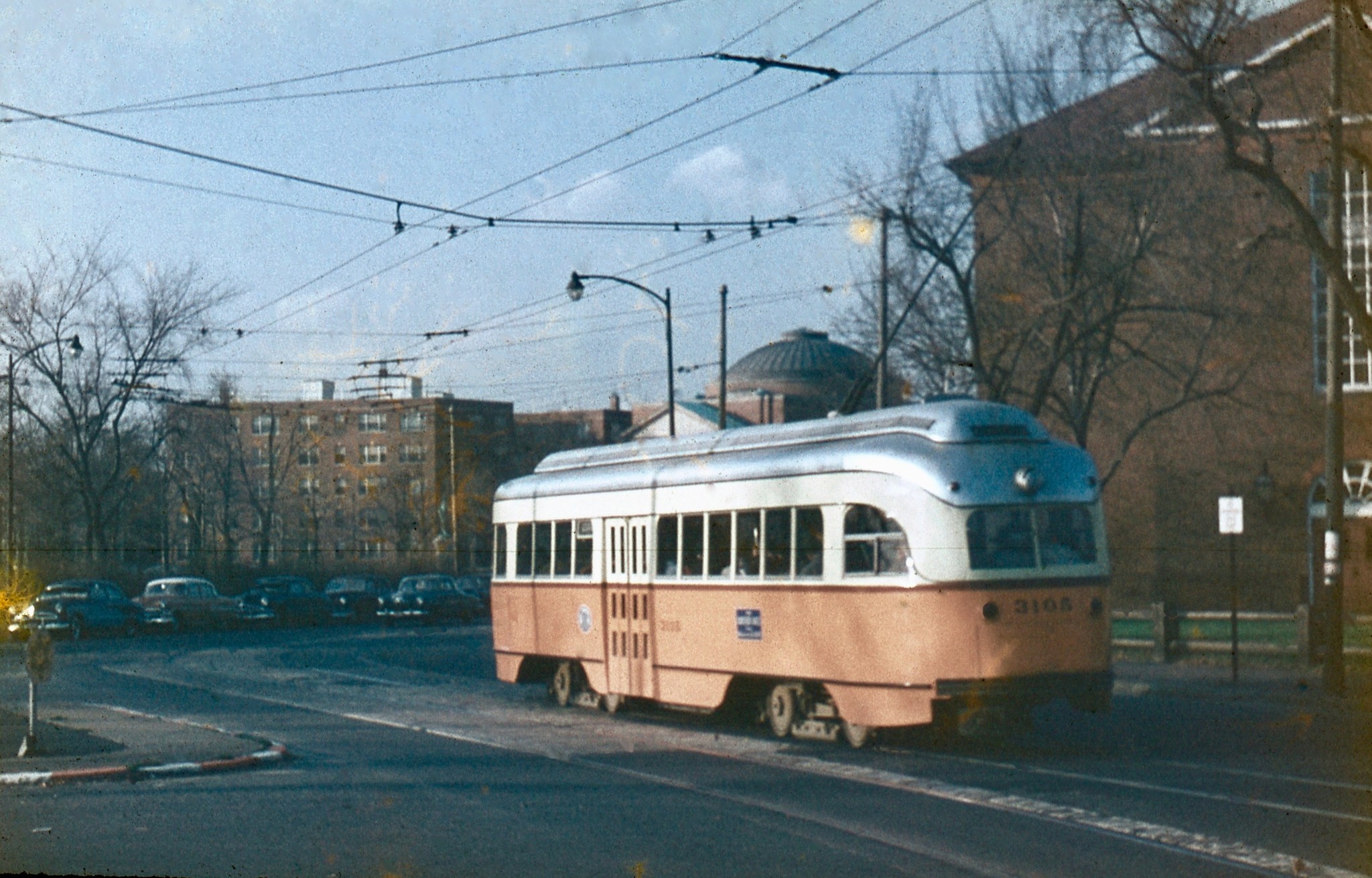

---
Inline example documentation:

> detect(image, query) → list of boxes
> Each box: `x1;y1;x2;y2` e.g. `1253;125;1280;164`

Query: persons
967;523;1086;570
515;534;593;576
659;510;906;575
164;584;190;627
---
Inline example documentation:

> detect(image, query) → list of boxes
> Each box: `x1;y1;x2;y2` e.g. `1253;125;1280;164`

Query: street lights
567;272;679;437
6;332;86;592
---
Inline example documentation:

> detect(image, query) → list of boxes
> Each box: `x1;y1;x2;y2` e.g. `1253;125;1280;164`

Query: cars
7;571;489;641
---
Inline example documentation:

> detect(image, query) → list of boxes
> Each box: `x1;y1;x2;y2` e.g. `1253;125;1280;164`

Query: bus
489;397;1115;751
